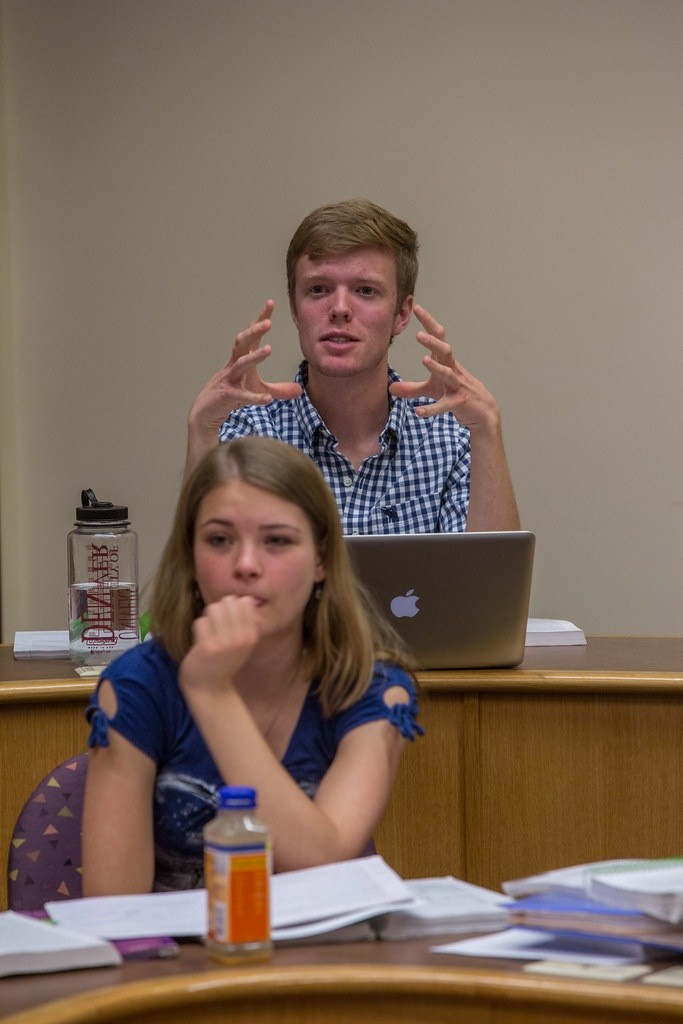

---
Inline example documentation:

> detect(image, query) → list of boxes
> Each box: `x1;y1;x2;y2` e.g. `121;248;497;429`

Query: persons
82;438;427;902
183;197;522;537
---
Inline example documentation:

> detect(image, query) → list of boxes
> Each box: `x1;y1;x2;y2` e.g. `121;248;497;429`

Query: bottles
202;786;273;967
67;489;138;667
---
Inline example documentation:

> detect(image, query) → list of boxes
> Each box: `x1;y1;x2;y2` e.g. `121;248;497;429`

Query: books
0;908;121;977
525;618;587;646
499;854;682;962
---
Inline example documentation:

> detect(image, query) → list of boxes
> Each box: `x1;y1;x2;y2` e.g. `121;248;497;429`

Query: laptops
343;531;536;671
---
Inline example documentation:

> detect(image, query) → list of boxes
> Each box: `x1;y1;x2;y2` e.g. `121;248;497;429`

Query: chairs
8;743;92;908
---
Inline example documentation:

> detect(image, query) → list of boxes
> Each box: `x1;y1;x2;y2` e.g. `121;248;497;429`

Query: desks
1;921;683;1024
0;639;683;911
